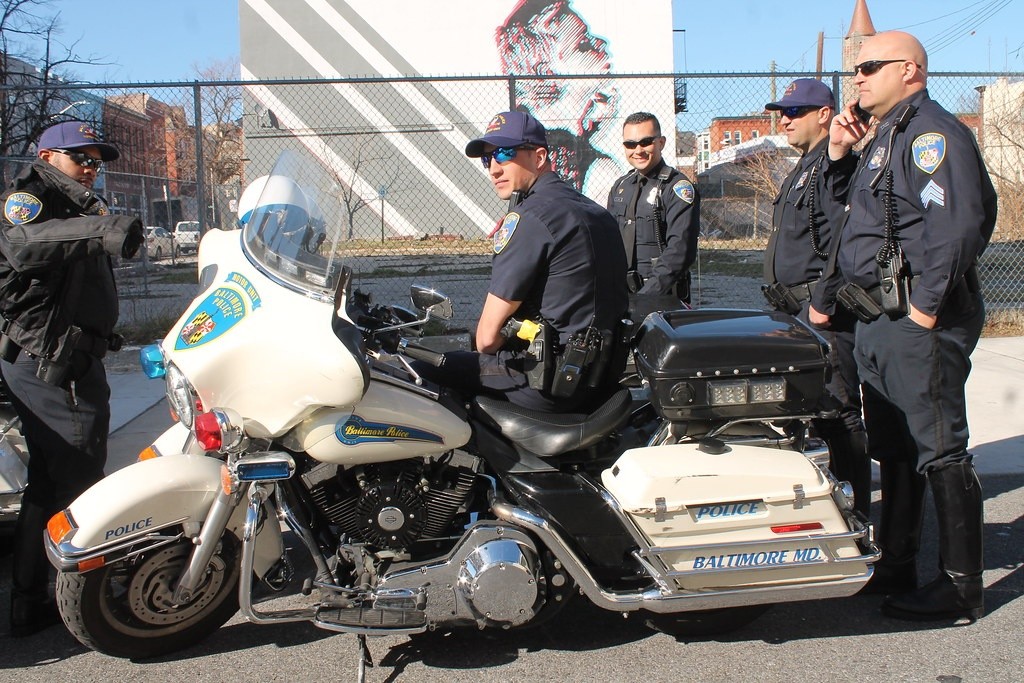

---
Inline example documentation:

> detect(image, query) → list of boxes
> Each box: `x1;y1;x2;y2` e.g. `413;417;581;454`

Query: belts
790;281;819;301
867;275;921;305
80;334;110;358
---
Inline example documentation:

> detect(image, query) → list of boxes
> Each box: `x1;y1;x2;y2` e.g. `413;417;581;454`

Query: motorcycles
42;144;885;683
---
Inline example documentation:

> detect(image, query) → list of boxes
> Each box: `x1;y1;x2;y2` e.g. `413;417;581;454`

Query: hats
36;121;120;163
765;78;835;110
465;110;549;158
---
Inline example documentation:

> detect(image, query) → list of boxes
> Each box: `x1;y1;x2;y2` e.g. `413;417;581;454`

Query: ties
762;159;802;286
621;175;646;271
822;126;879;282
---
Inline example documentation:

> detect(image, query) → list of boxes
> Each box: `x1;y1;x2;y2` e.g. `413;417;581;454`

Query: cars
132;225;181;261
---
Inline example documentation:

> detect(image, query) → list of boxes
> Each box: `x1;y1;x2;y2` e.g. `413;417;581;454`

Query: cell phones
852;103;873;124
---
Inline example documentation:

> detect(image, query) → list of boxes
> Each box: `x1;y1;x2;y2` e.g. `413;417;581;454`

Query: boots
9;498;64;638
830;432;870;522
854;472;926;597
881;464;986;621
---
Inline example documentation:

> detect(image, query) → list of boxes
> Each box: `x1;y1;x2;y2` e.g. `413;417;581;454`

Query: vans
174;221;209;254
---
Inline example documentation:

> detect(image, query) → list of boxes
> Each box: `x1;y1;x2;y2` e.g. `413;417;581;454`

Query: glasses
622;136;662;149
781;107;809;116
50;149;104;174
853;60;921;77
480;145;538;169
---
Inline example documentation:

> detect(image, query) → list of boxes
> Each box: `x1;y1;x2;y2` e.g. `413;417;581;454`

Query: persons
402;111;628;412
607;112;701;330
766;78;874;523
0;121;145;638
822;30;998;619
